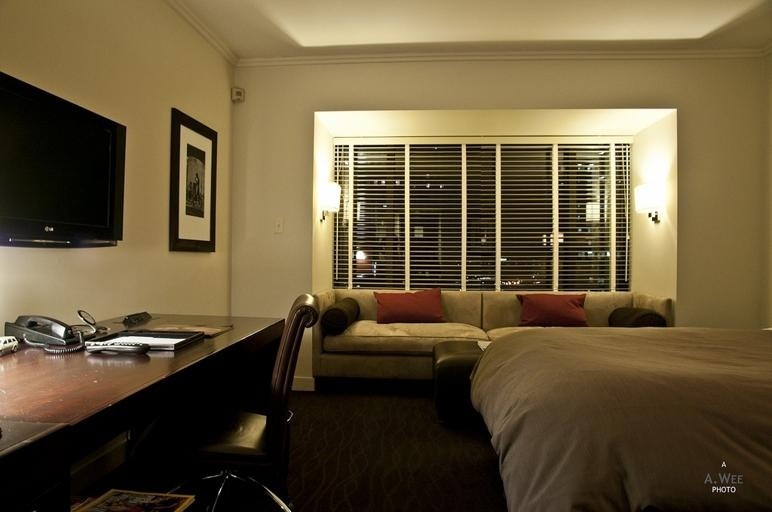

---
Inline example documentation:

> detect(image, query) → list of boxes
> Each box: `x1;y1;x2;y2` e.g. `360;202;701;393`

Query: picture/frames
169;108;219;253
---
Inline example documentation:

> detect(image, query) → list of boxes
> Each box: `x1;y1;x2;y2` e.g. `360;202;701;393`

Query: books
75;487;197;512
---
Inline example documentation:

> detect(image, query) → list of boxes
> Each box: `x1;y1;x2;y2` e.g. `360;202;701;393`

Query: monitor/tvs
0;71;127;248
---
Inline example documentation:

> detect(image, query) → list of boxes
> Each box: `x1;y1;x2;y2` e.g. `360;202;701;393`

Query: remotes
86;343;151;354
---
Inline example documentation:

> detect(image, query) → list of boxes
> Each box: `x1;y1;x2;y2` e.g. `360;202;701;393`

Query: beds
471;326;772;511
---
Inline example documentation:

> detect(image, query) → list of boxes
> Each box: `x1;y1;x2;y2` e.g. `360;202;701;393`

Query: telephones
5;316;96;345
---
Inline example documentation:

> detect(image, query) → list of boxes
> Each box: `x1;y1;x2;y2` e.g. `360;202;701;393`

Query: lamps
321;182;341;220
634;186;661;223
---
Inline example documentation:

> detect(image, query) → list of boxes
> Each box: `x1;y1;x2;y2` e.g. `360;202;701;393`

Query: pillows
516;294;588;324
373;288;446;322
608;307;667;326
319;297;359;335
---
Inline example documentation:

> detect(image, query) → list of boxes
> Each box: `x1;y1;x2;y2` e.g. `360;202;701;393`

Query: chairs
187;294;320;512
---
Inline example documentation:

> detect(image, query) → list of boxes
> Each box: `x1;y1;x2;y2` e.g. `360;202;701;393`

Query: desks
0;312;285;512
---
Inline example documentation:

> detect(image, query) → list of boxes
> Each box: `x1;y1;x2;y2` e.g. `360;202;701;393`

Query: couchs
312;289;671;379
